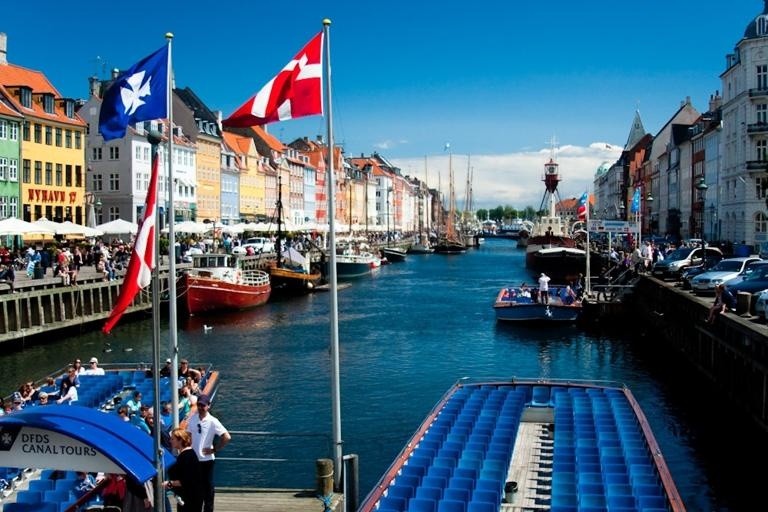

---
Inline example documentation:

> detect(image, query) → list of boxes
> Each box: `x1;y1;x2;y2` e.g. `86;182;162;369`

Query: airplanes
492;134;594;333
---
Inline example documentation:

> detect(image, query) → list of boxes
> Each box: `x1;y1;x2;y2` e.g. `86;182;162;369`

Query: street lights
618;200;627;221
694;175;710;272
645;192;656;243
95;197;104;225
602;208;608;219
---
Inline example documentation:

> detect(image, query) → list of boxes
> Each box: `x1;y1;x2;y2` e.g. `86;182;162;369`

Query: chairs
375;384;672;512
0;370;185;511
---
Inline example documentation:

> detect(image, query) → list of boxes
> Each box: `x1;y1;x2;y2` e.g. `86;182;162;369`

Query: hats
197;395;210;406
38;392;48;398
182;359;188;363
166;358;171;363
90;357;98;363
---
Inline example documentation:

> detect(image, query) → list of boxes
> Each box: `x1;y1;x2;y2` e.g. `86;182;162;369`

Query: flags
100;151;159;335
97;41;167;143
630;188;641;213
220;30;324;130
576;192;588;221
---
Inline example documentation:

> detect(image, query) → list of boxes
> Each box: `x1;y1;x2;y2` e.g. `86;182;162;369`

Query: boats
354;374;687;511
0;356;224;511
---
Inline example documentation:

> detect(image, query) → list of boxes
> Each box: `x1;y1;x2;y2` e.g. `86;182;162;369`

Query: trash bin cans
34;267;44;279
737;292;760;316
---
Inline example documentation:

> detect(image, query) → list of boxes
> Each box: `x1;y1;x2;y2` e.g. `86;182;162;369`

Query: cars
586;230;768;326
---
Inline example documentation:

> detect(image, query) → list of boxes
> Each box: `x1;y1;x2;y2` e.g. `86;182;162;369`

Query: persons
609;269;620;301
71;472;153;512
1;353;207;454
161;231;277;263
710;284;738;325
595;265;609;302
702;283;724;324
502;280;578;304
619;235;702;279
283;232;329;250
575;272;585;300
538;273;551;305
354;231;396;256
0;239;134;287
160;426;206;511
184;393;233;512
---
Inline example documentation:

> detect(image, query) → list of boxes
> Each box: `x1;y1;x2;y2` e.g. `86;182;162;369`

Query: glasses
198;424;201;433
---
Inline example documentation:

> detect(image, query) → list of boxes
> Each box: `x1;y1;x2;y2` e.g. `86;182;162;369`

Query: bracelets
167;480;173;490
